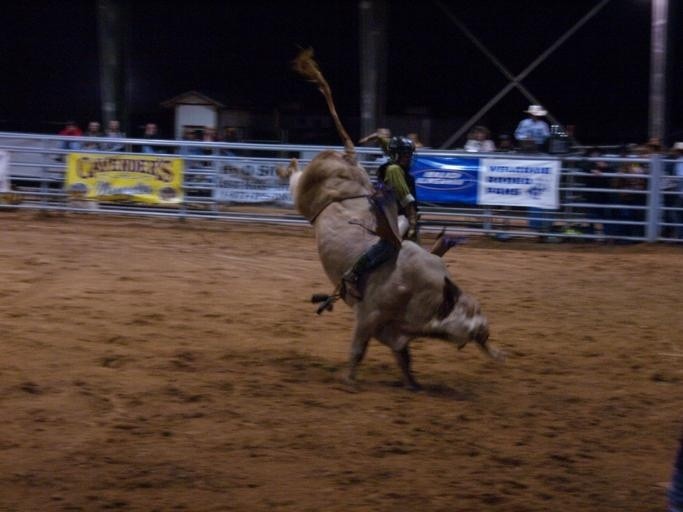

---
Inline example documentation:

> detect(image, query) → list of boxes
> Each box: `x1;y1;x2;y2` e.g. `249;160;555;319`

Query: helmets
387;136;415;169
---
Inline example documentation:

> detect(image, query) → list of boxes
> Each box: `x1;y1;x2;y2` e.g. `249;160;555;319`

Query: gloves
406;216;417;240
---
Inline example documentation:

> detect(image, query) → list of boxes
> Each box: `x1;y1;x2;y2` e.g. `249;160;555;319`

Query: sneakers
343;280;362;299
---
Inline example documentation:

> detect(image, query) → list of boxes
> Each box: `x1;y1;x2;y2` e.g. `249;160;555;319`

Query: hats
647;138;660;146
672;142;683;151
522;105;549;116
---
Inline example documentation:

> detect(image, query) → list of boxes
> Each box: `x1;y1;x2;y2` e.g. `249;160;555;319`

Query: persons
60;121;125;152
342;136;423;300
377;127;421;148
179;129;235;197
140;123;161;154
532;138;682;243
465;105;549;153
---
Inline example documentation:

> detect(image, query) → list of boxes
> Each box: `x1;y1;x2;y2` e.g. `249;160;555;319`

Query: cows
273;47;520;396
